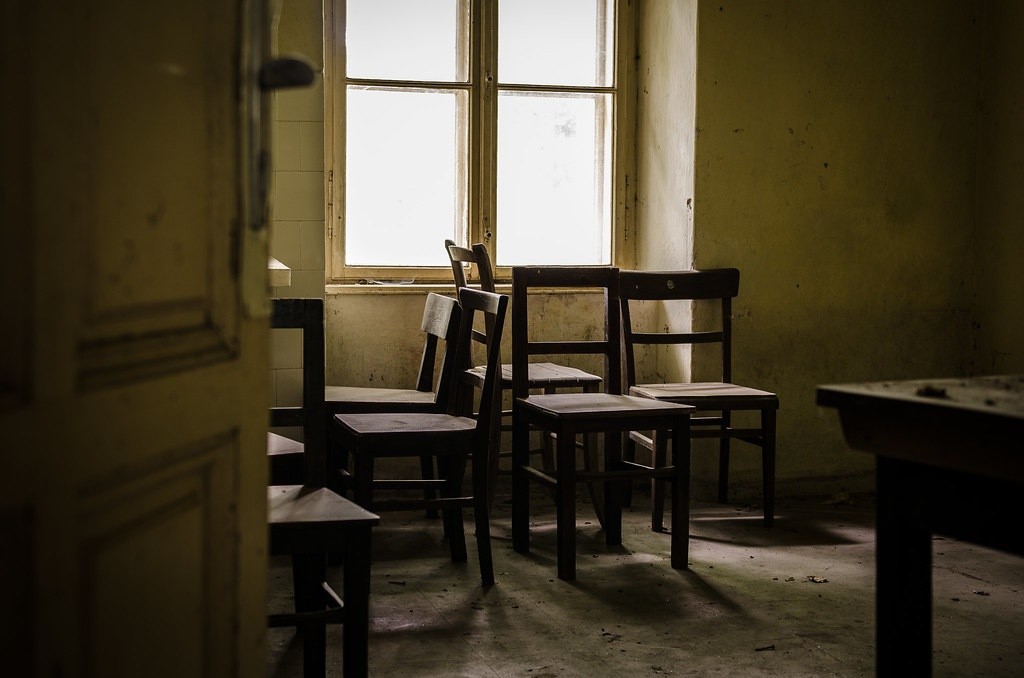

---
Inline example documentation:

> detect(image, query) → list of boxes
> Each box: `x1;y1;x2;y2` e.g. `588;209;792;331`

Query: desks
816;375;1024;678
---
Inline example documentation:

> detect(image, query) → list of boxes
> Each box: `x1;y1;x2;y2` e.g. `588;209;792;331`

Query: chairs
618;267;779;534
445;240;602;537
267;240;508;678
511;267;696;585
